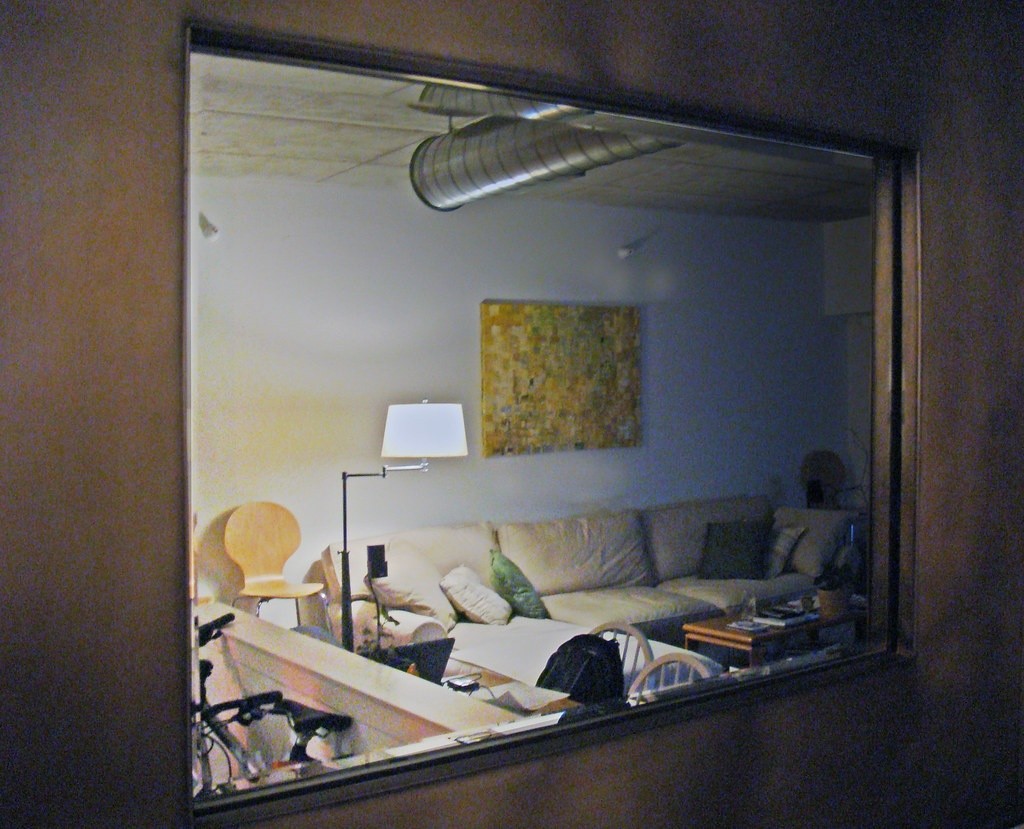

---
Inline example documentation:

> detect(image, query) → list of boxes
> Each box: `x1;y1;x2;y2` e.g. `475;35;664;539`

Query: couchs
318;494;866;650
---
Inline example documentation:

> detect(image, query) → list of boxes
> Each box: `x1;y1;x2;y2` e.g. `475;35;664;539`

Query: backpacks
535;633;625;706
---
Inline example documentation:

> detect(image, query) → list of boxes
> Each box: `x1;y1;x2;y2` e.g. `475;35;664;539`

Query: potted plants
814;554;855;617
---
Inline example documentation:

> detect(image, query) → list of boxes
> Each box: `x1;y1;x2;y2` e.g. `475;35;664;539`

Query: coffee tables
678;595;867;666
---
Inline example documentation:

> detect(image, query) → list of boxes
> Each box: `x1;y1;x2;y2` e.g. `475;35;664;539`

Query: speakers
807;478;825;505
367;546;389;580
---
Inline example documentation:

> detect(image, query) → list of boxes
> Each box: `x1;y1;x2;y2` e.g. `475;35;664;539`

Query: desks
443;619;725;695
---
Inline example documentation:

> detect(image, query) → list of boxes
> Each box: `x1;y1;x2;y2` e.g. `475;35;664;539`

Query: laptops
359;637;456;683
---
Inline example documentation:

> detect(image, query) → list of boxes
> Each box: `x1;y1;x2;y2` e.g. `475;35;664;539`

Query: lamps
338;402;469;650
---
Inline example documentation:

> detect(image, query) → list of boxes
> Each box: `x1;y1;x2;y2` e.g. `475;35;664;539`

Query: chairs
593;620;655;699
223;501;333;637
628;653;719;698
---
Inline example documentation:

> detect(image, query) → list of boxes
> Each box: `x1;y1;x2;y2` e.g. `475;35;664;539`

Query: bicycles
189;610;361;803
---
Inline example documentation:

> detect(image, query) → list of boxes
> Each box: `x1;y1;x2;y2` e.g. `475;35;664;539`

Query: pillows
490;549;551;621
441;564;514;626
695;515;776;579
362;549;459;633
763;523;811;577
